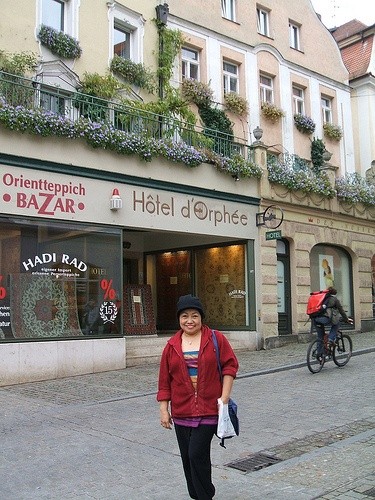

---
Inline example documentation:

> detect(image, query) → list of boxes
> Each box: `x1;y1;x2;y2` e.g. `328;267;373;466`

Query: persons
156;294;239;500
312;287;352;361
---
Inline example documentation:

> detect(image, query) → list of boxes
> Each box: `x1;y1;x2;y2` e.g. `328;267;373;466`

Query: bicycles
306;315;354;374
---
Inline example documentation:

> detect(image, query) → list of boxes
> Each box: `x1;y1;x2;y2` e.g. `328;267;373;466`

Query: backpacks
307;289;334;316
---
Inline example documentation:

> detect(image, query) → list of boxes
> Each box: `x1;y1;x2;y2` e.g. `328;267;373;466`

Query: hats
176;295;205;320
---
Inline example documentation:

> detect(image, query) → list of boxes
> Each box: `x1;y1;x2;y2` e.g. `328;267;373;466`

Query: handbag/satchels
215;396;238;440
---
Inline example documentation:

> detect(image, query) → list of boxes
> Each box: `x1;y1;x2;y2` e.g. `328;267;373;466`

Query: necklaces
187;339;192;344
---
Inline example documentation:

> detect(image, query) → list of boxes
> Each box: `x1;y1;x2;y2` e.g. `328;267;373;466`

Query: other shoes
327;339;338;346
